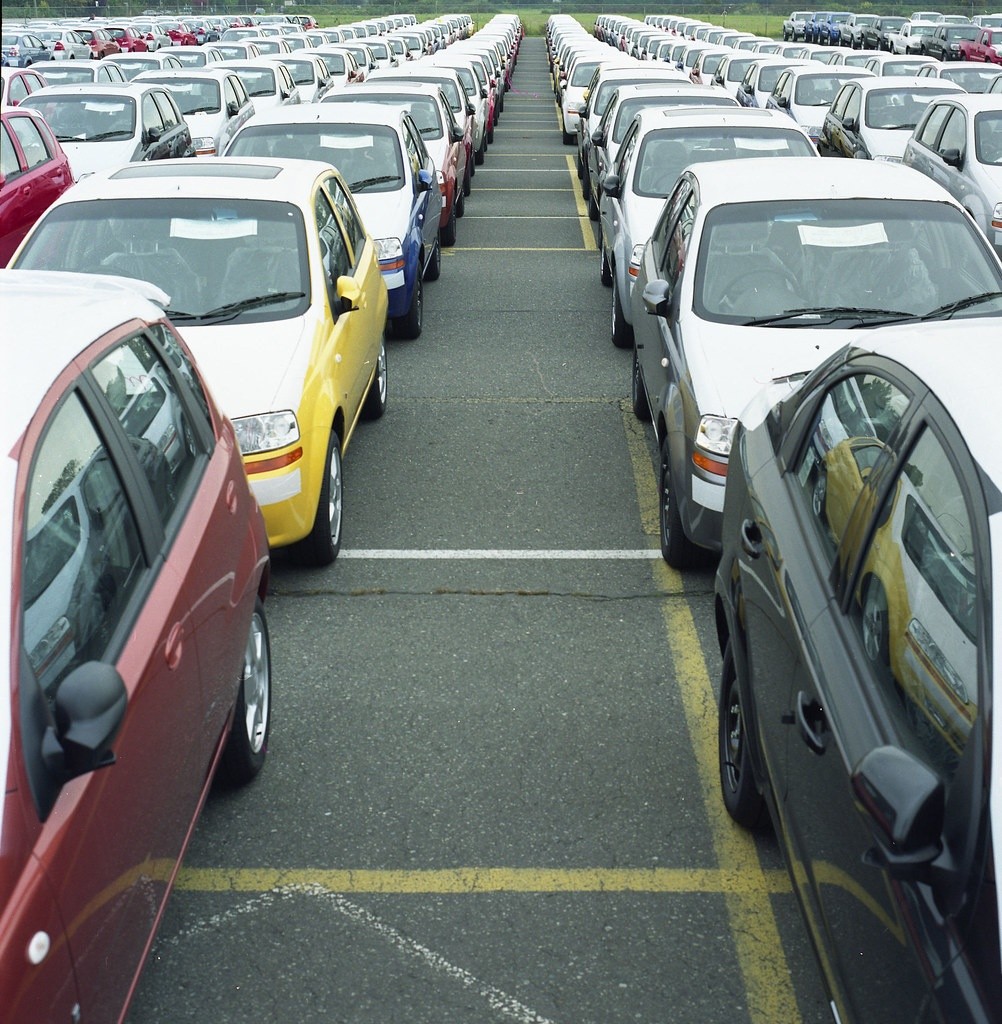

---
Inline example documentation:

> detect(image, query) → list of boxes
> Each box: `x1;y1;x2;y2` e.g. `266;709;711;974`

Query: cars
905;92;1000;250
104;52;196;98
1;265;275;1024
132;67;256;161
4;157;389;568
1;66;59;129
631;157;1000;568
0;32;55;68
16;81;197;219
804;434;985;750
596;107;834;352
0;0;1002;246
228;102;447;343
0;108;74;267
27;59;130;113
820;77;971;187
765;65;895;163
74;27;123;61
158;46;239;85
33;28;96;61
585;83;745;221
19;352;211;748
713;315;1002;1024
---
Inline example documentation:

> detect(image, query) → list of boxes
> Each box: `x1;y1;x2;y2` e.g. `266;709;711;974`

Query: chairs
704;34;996;157
831;219;936;313
249;76;273;95
107;106;131;136
411;108;437;135
643;141;689;193
97;218;199;313
209;221;303;307
331;57;344;72
195;84;217;109
705;210;803;314
272;137;308;159
294;65;312;83
55;107;93;138
337;133;379;182
